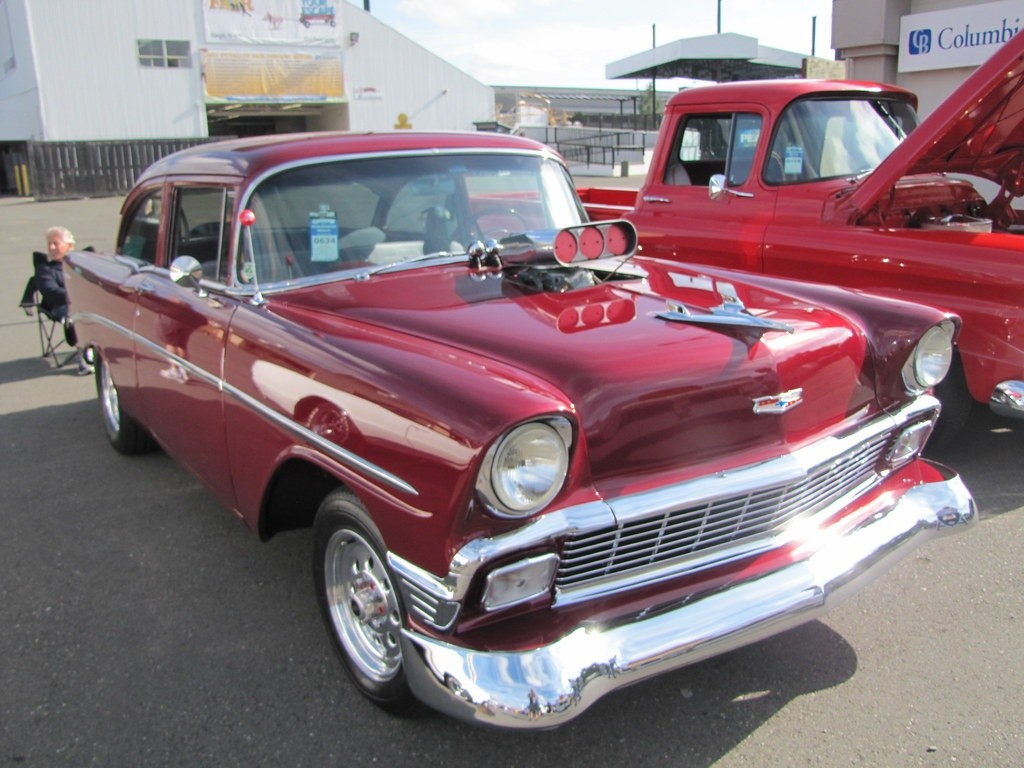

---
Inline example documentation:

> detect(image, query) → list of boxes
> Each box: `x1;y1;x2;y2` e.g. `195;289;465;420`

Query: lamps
351;33;360;46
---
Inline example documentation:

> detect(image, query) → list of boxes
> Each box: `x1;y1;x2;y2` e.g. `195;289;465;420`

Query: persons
155;302;208;382
37;226;94;364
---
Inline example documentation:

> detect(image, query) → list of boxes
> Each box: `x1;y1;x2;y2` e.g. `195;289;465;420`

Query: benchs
185;231;408;278
671;151;810;187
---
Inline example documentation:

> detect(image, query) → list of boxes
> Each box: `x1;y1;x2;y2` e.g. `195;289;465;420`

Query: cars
62;129;981;733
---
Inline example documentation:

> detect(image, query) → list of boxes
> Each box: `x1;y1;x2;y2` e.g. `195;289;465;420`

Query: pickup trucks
466;25;1023;426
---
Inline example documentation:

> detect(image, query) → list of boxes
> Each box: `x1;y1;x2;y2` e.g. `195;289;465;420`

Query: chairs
19;247;97;368
